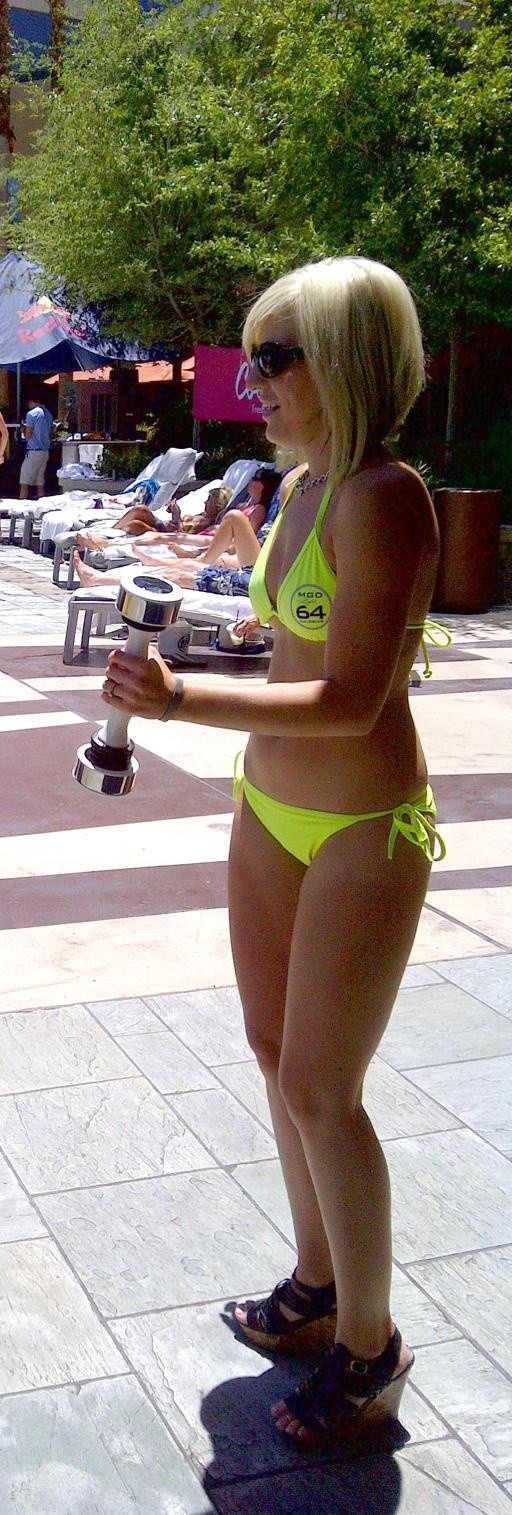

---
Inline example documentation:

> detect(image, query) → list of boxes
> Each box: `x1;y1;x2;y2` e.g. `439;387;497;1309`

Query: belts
29;449;42;450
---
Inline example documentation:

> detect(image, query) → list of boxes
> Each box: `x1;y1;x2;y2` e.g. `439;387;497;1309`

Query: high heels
237;1268;338;1355
273;1328;414;1452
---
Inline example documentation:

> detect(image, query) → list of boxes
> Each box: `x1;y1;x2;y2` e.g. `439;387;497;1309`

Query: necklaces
295;470;330;499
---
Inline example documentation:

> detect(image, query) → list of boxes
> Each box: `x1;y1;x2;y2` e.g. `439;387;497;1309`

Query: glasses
251;341;306;379
252;477;261;481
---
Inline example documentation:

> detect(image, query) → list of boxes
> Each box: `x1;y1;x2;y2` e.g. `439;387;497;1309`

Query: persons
15;419;28;441
99;255;442;1447
73;467;281;639
17;397;54;499
1;411;11;467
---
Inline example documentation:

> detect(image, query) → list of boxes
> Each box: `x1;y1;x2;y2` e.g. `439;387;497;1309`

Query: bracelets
158;678;183;723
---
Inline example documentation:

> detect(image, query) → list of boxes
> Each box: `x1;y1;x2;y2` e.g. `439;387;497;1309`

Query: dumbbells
72;573;186;797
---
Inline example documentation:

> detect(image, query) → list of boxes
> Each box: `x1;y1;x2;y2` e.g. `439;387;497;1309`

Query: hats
81;433;104;439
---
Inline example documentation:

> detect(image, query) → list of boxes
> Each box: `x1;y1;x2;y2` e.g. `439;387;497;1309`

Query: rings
109;682;117;697
